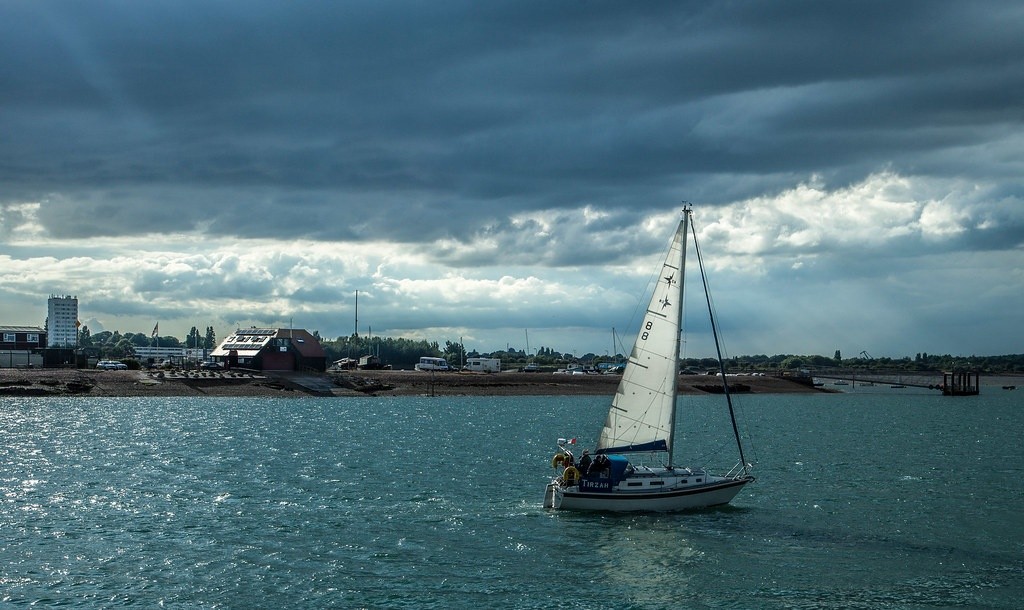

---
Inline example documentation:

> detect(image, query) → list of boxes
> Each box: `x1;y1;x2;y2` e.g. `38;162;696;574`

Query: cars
553;366;624;377
524;366;542;373
705;370;766;376
96;361;116;371
448;365;459;373
679;370;698;376
202;362;223;371
110;361;128;370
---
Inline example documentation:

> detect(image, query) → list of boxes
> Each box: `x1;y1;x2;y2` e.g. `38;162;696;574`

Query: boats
813;378;824;386
834;379;848;385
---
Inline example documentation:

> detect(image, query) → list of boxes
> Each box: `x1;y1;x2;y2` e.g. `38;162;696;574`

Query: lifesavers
552;454;565;470
563;466;579;483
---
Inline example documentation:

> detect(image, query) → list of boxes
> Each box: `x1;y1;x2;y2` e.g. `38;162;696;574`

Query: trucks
463;358;501;374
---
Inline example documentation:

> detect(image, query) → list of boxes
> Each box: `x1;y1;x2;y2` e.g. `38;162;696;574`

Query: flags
151;322;159;336
75;320;81;327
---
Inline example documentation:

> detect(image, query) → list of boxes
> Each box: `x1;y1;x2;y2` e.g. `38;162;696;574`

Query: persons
587;455;608;482
577;448;592;479
562;451;574;479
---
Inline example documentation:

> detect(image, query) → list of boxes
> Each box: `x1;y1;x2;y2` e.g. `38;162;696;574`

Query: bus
420;357;448;372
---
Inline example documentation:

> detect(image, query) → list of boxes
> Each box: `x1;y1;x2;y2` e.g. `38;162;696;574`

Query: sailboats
547;202;755;512
